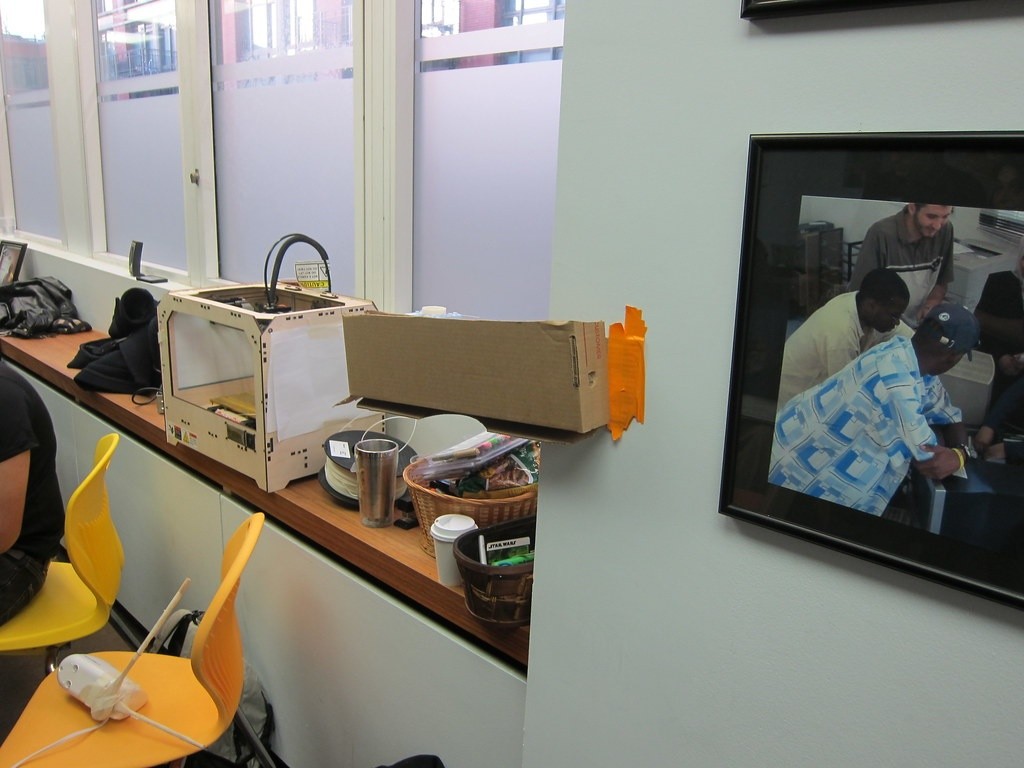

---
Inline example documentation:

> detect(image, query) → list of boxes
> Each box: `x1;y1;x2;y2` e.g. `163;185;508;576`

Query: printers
943;239;1020;314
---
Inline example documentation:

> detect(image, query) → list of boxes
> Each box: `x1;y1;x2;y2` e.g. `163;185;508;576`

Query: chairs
0;433;125;678
0;510;267;768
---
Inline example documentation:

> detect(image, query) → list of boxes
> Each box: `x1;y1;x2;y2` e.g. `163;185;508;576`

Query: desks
1;331;531;767
912;428;1024;553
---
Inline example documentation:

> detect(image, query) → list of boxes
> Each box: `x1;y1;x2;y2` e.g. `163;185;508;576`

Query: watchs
959;443;971;464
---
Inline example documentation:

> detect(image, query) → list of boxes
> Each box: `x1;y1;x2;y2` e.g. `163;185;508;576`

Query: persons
1;356;67;632
767;200;1023;568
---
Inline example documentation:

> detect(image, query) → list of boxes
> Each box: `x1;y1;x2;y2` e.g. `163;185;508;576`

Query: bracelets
951;447;965;473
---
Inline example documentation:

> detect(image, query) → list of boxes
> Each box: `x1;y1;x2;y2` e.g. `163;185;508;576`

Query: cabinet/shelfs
791;227;843;317
817;227;865;308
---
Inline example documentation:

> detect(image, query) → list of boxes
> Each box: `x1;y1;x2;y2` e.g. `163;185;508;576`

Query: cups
354;438;398;528
430;514;478;587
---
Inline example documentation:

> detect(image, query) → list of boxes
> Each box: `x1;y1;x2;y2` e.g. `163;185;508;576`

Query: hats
922;301;980;362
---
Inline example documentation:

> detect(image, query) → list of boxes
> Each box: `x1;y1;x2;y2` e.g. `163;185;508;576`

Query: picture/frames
0;240;28;287
738;1;947;21
716;130;1024;611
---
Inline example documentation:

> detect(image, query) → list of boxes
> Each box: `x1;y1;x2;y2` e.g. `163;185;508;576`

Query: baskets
403;457;538;559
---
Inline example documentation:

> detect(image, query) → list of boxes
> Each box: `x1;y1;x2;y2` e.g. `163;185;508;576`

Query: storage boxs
341;309;612;444
452;515;537;627
